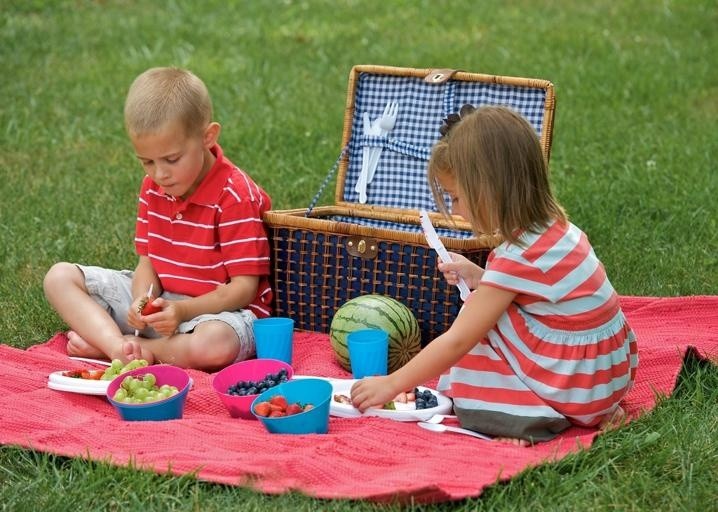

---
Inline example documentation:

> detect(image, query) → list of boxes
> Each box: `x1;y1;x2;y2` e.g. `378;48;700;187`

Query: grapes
415;387;438;410
100;359;179;404
226;368;288;396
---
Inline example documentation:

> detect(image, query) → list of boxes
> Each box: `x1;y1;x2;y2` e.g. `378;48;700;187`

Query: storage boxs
262;63;558;349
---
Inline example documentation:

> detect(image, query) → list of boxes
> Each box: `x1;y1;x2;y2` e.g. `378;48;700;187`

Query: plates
47;369;194;396
290;376;455;422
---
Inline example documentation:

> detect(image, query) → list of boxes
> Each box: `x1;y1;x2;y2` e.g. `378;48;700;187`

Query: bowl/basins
105;364;195;420
212;359;294;421
250;378;333;434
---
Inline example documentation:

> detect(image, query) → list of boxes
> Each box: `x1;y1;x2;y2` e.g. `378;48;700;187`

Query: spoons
417;421;495;442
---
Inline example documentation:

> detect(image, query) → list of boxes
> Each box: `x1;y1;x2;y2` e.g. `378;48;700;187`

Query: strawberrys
137;297;161;316
254;396;314;417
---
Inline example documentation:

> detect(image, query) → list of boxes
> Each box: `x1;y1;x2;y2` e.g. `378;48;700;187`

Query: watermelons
329;294;422;376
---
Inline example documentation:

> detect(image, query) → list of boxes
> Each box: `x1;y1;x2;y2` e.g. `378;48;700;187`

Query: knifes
418;206;471;302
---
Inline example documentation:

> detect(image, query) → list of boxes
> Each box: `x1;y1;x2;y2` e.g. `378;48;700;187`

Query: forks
355;100;400;204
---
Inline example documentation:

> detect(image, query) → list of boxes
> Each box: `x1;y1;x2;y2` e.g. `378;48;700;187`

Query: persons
349;104;641;448
43;67;274;376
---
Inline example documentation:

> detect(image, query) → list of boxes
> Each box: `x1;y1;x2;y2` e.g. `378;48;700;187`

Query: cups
253;317;296;366
347;328;389;379
